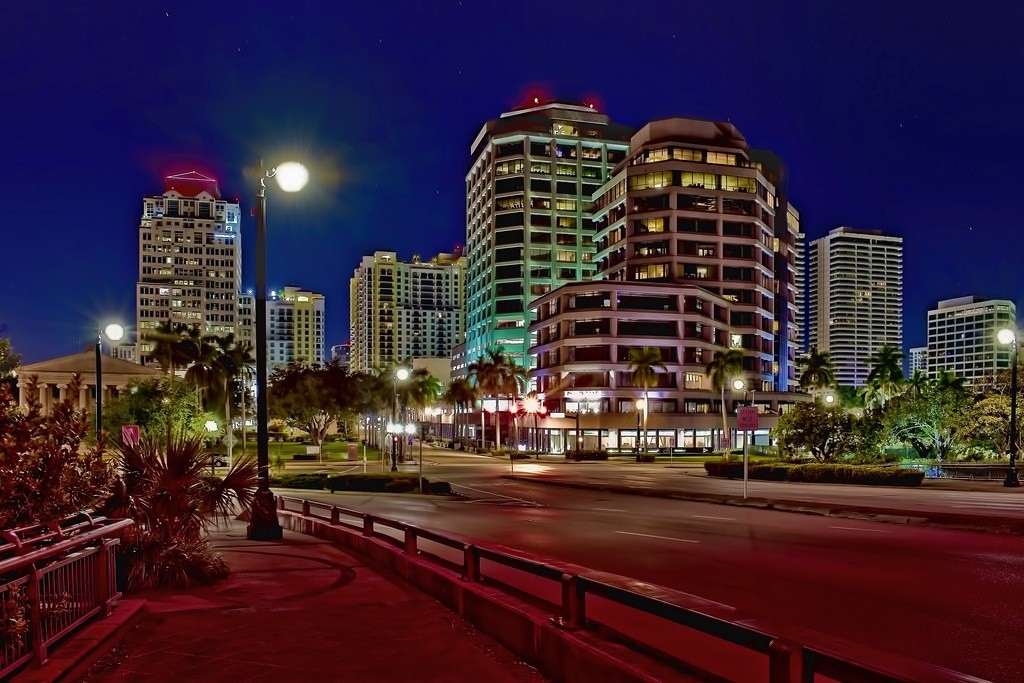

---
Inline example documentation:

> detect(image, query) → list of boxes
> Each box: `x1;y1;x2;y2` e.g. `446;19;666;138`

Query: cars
191;451;231;467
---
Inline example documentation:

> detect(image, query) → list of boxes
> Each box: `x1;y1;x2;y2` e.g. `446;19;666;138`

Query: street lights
94;323;124;456
525;399;540;458
731;378;748;464
391;369;409;472
997;326;1021;489
244;155;311;541
825;392;835;458
636;399;645;456
